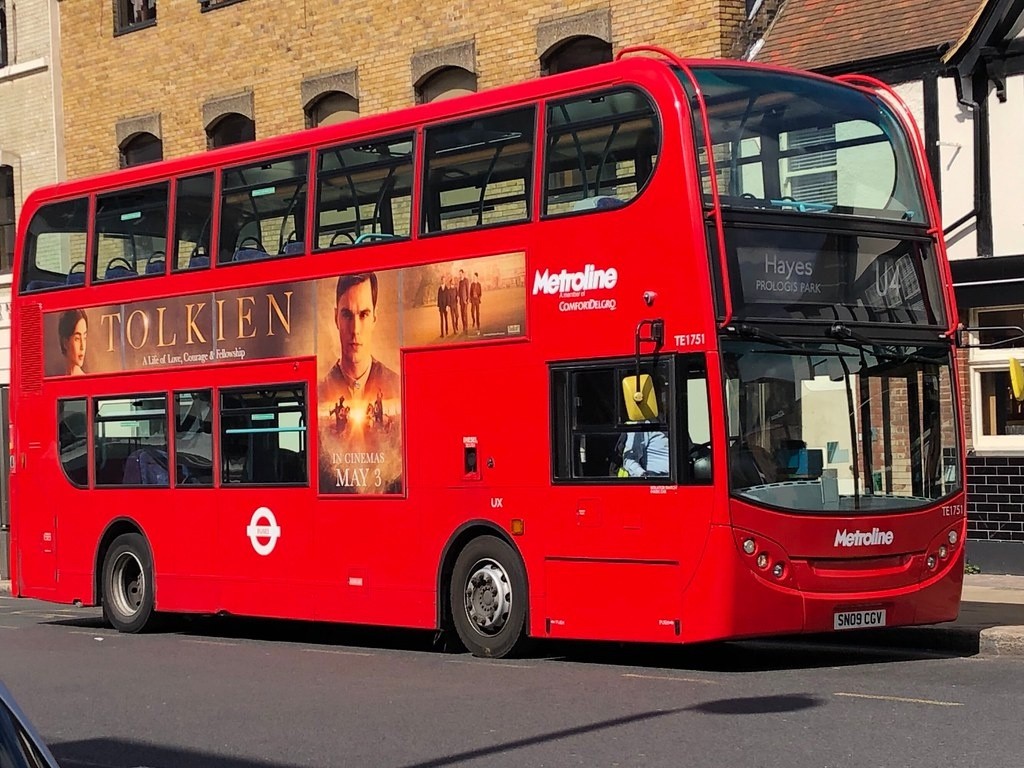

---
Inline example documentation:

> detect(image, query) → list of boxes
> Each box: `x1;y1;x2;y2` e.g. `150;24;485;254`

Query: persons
320;386;395;438
437;275;450;338
457;268;471;336
446;276;461;336
620;383;671;478
58;308;89;376
469;272;483;336
727;419;779;492
318;271;402;405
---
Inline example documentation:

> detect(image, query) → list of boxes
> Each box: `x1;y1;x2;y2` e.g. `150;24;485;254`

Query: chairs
188;246;210;268
104;257;137;280
123;449;188;484
329;232;354;248
26;279;65;291
231;237;271;262
286;230;304;255
144;251;166;275
66;261;86;285
572;196;624;212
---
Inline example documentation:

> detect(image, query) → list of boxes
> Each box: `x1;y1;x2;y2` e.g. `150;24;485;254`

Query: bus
6;42;1024;660
